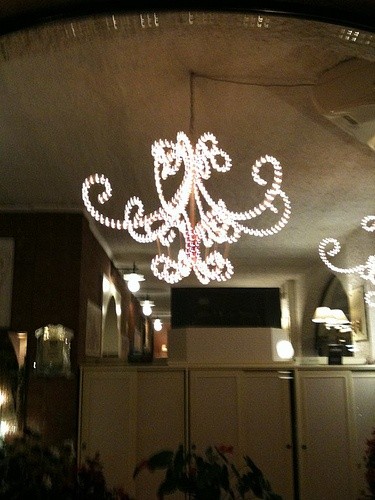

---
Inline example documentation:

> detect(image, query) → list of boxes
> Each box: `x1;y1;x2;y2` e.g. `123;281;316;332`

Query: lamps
123;261;146;293
161;343;168;352
318;215;375;308
312;307;361;335
139;295;156;316
153;316;162;332
80;71;291;285
